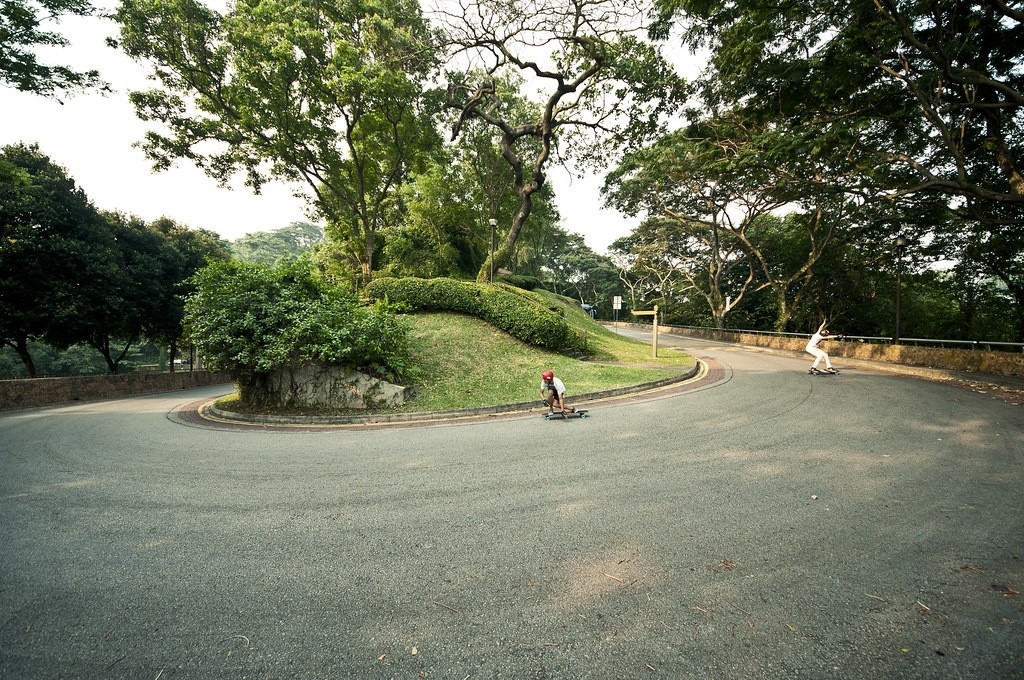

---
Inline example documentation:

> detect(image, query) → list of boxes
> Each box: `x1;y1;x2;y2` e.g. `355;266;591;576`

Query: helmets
821;329;829;335
542;371;554;381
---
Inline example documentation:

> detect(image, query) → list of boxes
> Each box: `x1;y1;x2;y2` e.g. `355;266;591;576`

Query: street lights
894;232;908;345
488;212;499;283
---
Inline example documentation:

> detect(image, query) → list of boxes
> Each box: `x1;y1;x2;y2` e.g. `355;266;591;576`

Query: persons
805;318;843;372
539;371;578;418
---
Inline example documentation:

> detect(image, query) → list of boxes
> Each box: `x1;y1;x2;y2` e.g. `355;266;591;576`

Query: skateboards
542;409;588;421
807;367;840;375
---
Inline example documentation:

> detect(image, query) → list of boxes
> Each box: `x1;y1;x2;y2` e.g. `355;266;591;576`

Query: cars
167;358;193;367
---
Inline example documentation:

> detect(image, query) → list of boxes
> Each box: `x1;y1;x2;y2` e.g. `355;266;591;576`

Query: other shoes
828;367;837;372
548;410;553;416
571;406;576;413
811;367;819;373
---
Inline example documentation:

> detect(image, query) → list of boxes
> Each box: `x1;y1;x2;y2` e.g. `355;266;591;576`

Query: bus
581;305;597;320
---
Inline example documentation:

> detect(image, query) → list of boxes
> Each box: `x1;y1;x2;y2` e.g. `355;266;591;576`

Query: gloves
543;398;548;407
562;410;567;417
